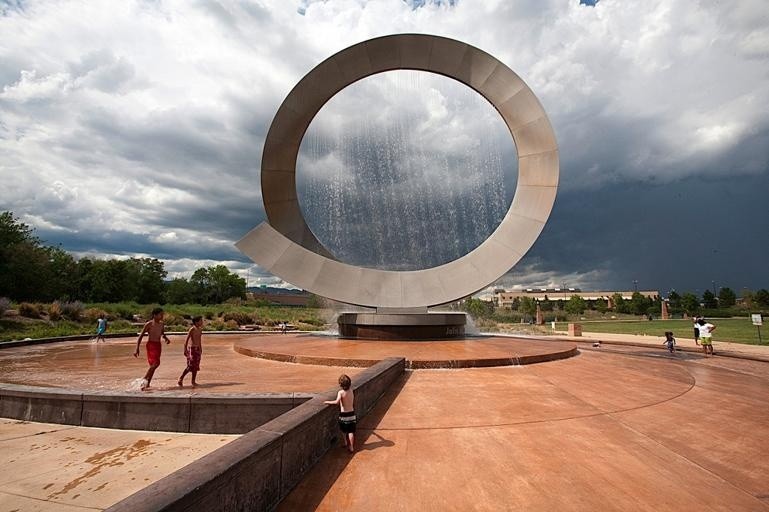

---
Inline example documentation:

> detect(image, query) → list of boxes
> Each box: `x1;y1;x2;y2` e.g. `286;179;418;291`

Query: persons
693;313;716;358
281;322;286;335
133;308;170;389
95;313;107;343
177;315;202;386
324;375;356;452
663;332;676;353
